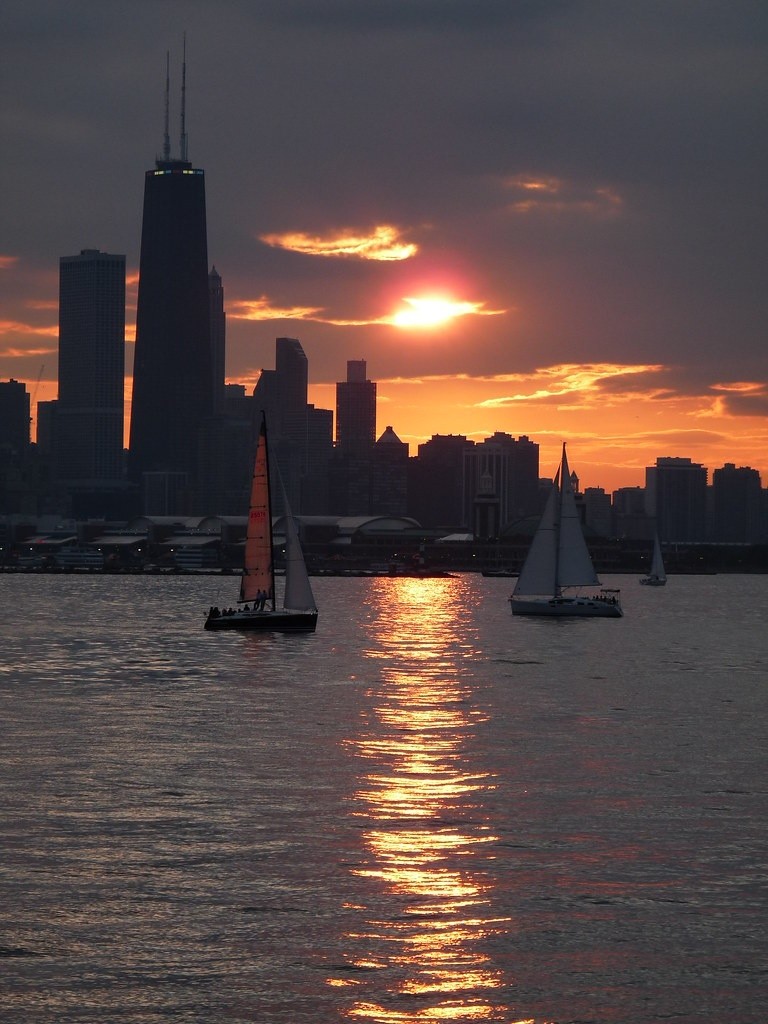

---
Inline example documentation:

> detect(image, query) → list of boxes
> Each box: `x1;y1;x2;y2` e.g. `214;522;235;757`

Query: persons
596;596;599;599
244;604;250;611
600;596;603;600
593;595;595;598
209;607;221;618
238;609;243;613
612;596;616;604
222;608;228;616
604;596;607;601
254;589;267;610
228;608;236;616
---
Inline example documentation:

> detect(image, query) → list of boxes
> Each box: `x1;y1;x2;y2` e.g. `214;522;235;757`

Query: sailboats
507;441;624;618
204;406;320;635
639;533;668;587
